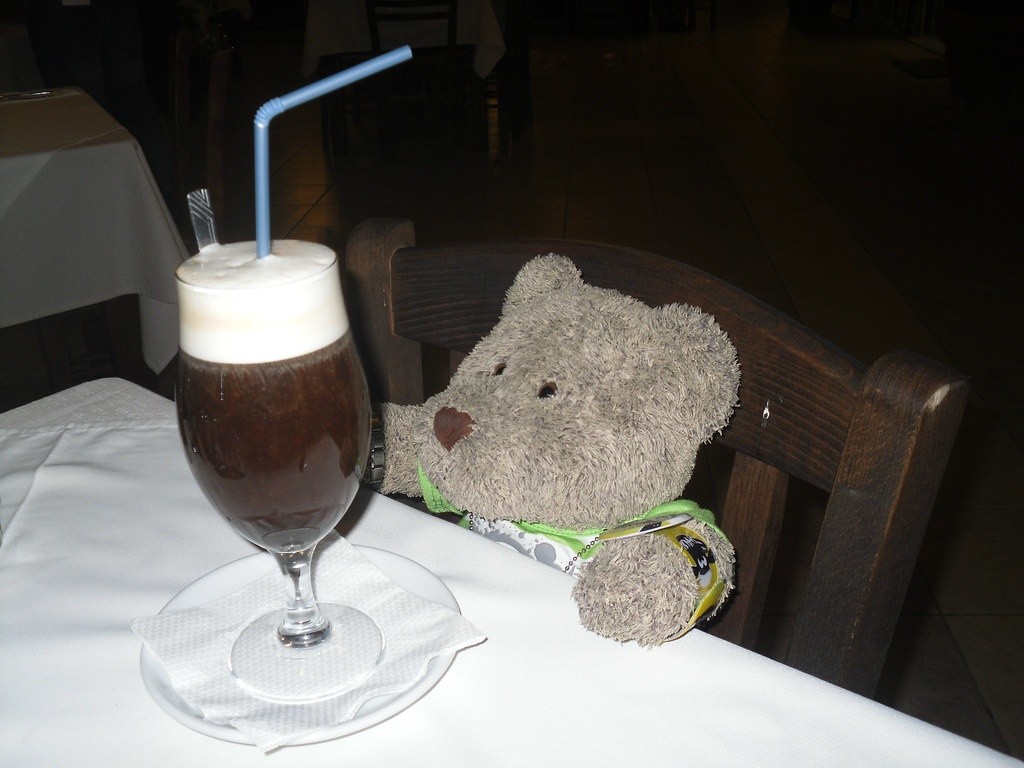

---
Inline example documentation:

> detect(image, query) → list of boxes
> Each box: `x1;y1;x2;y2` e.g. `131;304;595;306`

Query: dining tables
1;86;193;382
0;377;1024;768
299;0;506;151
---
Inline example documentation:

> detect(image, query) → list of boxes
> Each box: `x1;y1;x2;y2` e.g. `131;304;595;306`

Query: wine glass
172;238;384;704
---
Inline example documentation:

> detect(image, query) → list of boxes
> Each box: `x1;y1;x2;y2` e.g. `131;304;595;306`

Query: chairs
34;22;231;394
362;0;474;163
341;215;980;700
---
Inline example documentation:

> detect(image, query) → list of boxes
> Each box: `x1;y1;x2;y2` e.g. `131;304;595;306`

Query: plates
139;542;465;744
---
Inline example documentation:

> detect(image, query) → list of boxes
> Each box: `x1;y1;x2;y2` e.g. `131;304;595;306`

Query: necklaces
467;508;599;574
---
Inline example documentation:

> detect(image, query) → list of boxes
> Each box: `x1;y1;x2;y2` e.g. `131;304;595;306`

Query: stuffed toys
368;253;741;649
